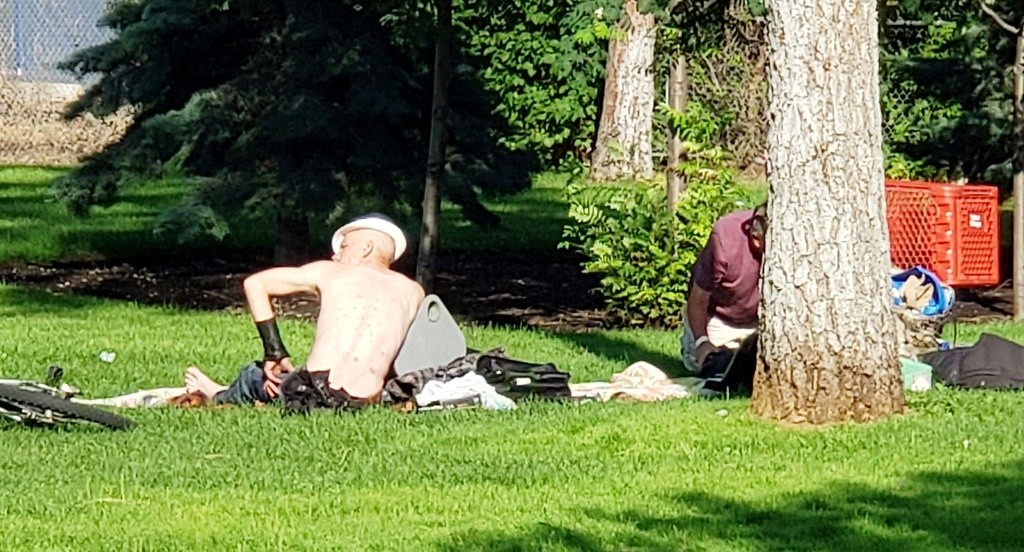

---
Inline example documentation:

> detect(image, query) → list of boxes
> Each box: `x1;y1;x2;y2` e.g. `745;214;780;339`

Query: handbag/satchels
474;355;572;399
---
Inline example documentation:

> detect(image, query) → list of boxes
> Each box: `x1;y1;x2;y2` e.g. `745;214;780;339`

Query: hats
331;213;407;262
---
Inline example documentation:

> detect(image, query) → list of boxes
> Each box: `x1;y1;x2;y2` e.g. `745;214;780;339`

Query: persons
184;212;426;407
680;203;767;374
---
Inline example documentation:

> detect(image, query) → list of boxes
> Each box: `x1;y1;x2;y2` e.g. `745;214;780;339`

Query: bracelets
695;335;708;349
